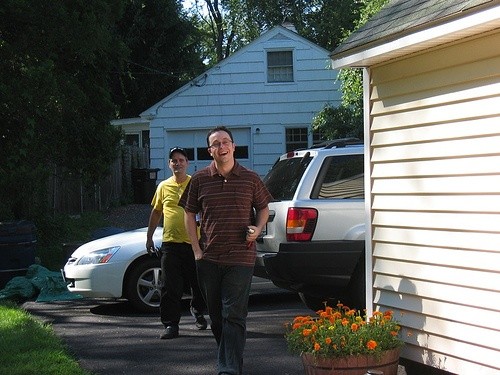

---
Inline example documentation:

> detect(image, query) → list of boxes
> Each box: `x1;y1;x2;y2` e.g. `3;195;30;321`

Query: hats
169;145;187;159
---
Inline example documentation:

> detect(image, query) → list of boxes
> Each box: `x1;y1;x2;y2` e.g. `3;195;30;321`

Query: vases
301;349;402;375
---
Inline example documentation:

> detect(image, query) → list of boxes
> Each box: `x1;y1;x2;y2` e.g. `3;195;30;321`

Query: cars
60;214;297;315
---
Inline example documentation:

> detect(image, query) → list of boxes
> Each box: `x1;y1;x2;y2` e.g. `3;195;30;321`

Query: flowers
283;302;417;364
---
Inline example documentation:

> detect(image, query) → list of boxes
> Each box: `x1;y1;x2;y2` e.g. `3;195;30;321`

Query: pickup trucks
252;137;368;314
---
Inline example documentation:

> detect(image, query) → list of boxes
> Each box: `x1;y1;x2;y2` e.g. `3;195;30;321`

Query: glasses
208;139;232;149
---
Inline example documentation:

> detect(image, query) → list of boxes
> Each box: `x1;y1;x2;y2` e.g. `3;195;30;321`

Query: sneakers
159;324;179;339
190;305;207;330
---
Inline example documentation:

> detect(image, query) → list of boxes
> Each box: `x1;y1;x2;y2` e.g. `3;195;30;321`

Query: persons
146;147;207;338
177;127;275;375
